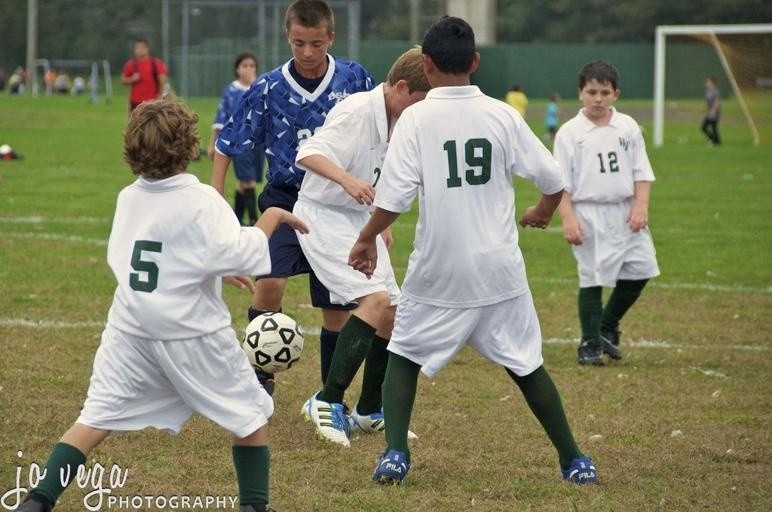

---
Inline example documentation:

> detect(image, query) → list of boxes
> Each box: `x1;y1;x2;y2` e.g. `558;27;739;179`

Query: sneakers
254;371;275;396
371;449;411;485
301;391;352;448
577;337;607;364
560;456;598;486
600;309;623;360
341;402;351;438
16;491;54;511
347;405;420;440
238;503;278;511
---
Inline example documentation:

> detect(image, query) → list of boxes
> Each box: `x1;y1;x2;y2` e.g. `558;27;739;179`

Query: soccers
240;310;306;375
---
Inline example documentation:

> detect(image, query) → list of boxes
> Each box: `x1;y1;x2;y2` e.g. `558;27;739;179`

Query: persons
292;43;432;446
0;65;84;94
504;85;528;127
207;0;379;422
556;60;661;365
548;93;562;139
702;77;722;145
17;101;308;506
208;52;260;228
348;15;598;483
122;40;169;109
